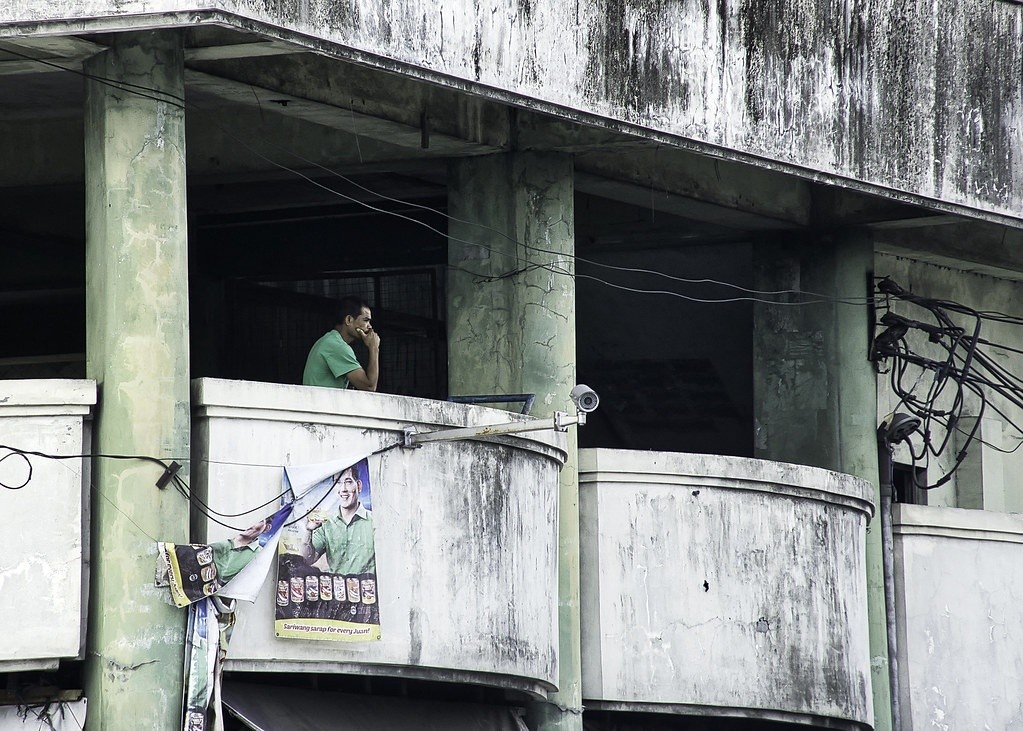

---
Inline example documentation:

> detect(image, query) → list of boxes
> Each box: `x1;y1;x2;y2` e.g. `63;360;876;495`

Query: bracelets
307;528;312;532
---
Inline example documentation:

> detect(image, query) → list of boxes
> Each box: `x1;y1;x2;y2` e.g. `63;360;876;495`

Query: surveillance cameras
570;384;599;413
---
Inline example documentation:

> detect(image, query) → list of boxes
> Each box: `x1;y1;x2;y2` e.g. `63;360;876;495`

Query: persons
300;466;376;575
304;296;381;391
191;516;273;591
